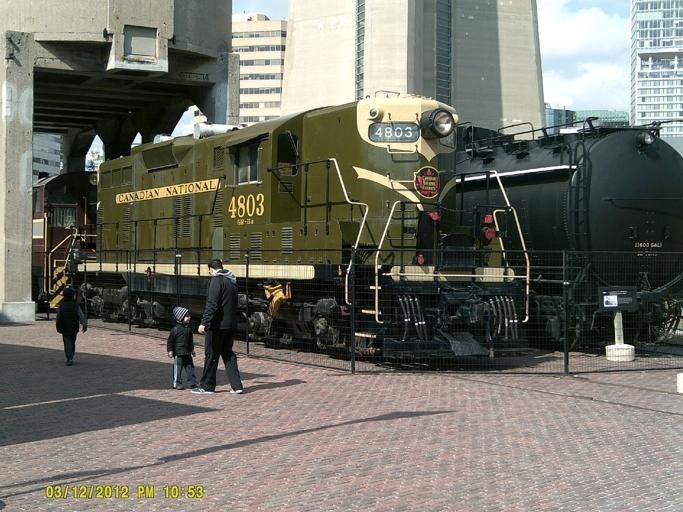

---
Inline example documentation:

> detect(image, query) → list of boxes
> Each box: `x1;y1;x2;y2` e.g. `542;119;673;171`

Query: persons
167;306;200;390
56;288;87;365
191;259;243;394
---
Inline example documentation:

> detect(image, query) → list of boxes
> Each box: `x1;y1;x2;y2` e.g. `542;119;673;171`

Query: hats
173;307;188;322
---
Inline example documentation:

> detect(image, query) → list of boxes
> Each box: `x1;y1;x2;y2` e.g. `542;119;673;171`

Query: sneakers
173;383;214;394
230;389;243;394
